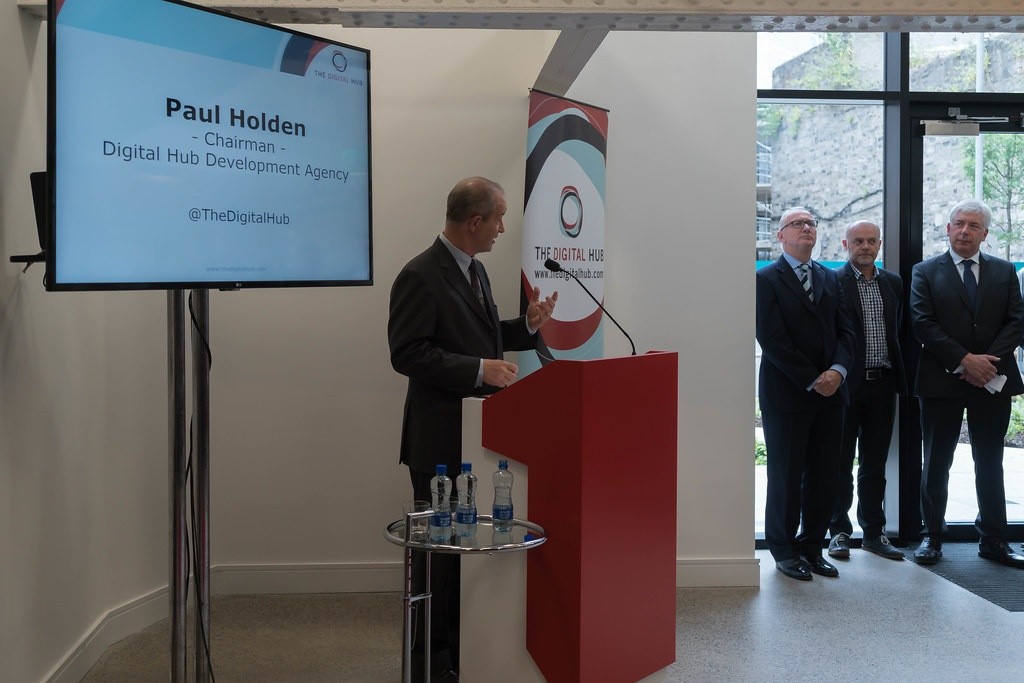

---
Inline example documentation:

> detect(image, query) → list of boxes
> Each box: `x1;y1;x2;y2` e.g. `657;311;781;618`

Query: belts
860;370;888;380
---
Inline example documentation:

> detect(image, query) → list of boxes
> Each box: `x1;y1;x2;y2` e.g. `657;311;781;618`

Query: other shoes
827;533;850;555
861;535;906;559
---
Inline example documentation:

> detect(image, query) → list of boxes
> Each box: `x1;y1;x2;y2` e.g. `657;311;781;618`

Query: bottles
493;460;514;532
455;462;478;537
429;464;452;540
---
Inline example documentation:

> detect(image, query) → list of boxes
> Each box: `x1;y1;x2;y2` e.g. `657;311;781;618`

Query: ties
960;260;978;309
469;259;491;323
801;264;815;303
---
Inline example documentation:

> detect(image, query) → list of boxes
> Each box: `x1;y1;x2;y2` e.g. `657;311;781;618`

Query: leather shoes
776;556;837;581
913;537;943;565
978;539;1024;568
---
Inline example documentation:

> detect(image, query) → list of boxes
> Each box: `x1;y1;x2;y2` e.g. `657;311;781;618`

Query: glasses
779;219;818;232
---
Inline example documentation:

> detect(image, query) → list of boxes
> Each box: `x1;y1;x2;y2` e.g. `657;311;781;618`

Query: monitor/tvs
45;0;374;292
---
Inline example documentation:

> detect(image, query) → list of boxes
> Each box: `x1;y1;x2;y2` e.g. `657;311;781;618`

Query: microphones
544;258;637;356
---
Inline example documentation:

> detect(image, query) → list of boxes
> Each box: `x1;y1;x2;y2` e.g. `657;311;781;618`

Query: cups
402;499;430;533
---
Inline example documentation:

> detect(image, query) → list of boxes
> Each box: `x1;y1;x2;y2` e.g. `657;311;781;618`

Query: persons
387;175;558;683
828;220;906;559
755;207;858;580
910;200;1024;570
